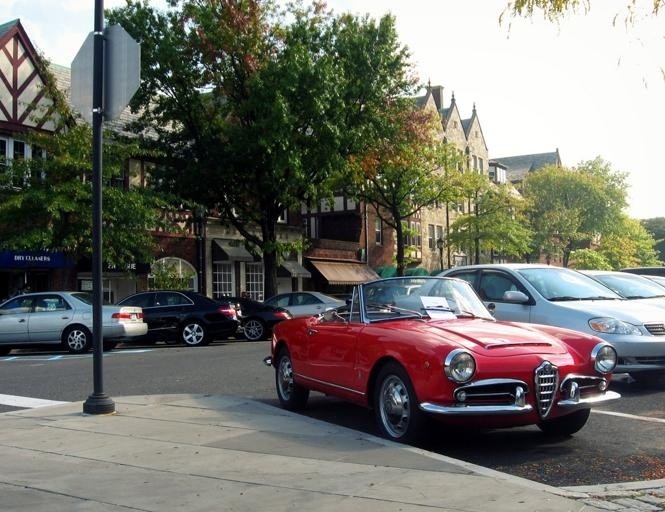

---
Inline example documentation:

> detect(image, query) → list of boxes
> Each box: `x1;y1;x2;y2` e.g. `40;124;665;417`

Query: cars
213;296;293;340
400;262;663;394
114;290;242;346
641;274;664;287
262;291;347;319
262;274;623;450
0;290;149;356
578;269;664;326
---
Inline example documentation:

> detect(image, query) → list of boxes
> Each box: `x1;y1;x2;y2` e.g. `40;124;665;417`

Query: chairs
294;297;313;305
481;280;500;300
33;301;64;312
147;298;163;306
